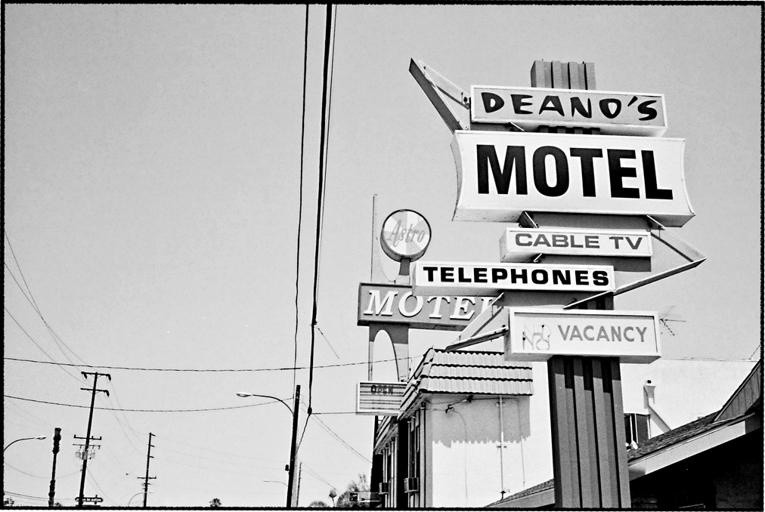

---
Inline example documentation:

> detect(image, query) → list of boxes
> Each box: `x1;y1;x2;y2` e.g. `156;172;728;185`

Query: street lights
236;392;299;508
4;435;46;452
